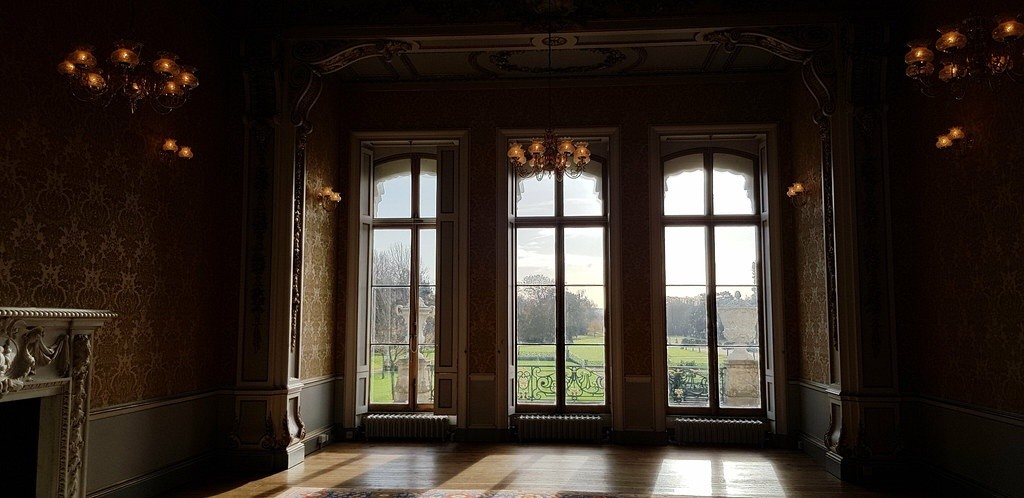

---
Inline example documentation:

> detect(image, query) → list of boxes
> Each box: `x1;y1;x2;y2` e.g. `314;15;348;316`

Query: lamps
786;182;812;209
157;123;195;167
935;126;974;158
506;0;592;184
53;0;200;113
903;0;1024;101
